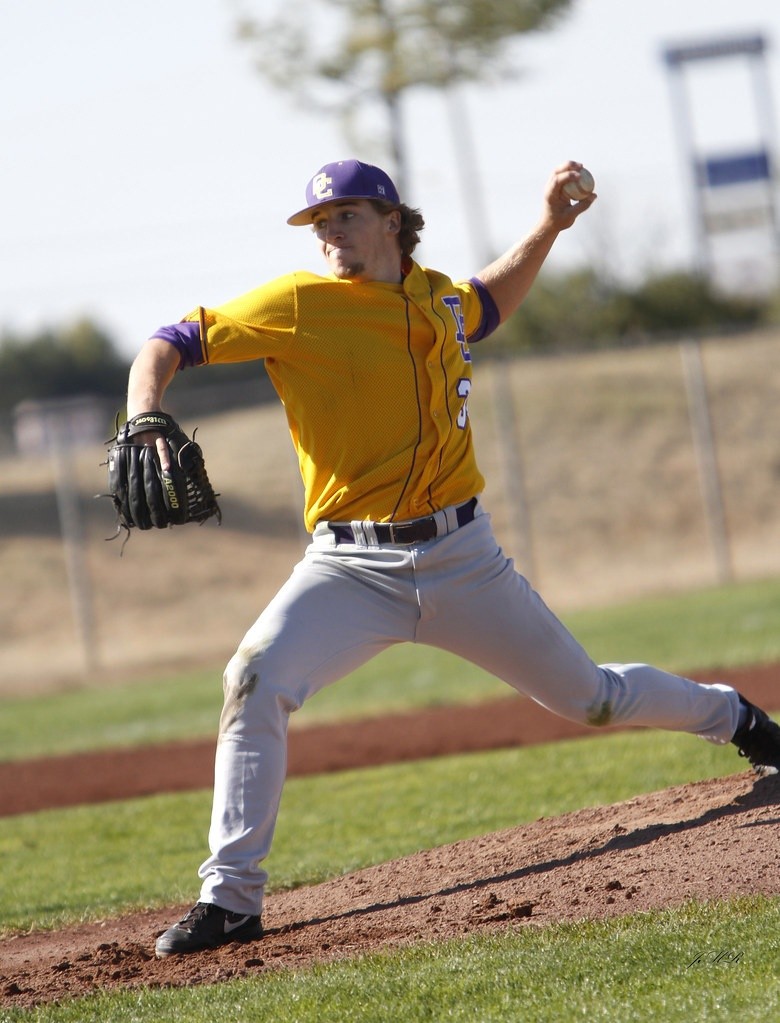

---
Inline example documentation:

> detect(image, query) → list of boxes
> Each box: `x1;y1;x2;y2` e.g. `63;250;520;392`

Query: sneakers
153;902;265;959
729;692;780;770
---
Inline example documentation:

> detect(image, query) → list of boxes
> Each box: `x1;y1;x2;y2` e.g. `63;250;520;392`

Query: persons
122;159;780;959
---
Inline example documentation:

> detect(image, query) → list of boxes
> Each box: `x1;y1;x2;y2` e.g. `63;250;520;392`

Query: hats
287;159;400;226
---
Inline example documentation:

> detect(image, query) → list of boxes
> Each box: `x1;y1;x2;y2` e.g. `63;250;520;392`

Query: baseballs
561;167;594;201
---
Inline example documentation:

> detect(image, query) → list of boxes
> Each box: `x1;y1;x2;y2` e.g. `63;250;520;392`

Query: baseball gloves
105;411;220;532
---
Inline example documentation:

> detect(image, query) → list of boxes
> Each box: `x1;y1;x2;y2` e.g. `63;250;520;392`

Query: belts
328;496;478;544
